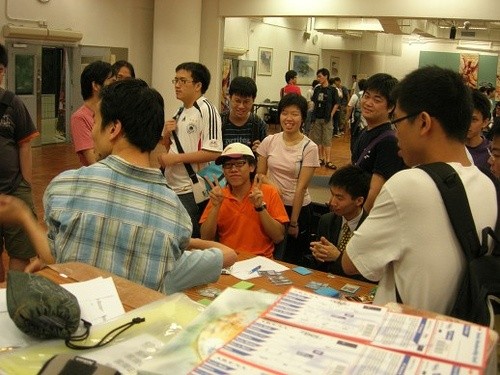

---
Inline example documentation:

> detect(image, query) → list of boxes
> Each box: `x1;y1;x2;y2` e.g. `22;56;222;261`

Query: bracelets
254;201;267;212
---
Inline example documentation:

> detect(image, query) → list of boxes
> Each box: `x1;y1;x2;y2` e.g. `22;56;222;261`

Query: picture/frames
288;50;320;86
258;46;274;77
330;56;341;78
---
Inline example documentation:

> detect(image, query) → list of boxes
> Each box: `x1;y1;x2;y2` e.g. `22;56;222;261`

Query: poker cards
342;283;377;304
304;280;329;290
257;269;294;286
197;285;221;298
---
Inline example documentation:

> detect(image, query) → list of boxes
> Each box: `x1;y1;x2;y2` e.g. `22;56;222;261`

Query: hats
214;142;254;165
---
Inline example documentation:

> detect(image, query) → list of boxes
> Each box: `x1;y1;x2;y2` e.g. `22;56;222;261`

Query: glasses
390;110;429;132
172;79;200;85
222;162;252;169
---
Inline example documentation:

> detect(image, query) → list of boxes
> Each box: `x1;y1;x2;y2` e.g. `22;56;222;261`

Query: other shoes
334;134;340;137
341;131;344;134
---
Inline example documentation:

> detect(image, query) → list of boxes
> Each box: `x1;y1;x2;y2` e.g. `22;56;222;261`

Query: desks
179;250;380;309
252;102;279;112
0;260;168;313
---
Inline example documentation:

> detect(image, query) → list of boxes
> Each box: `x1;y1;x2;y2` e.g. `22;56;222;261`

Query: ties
339;221;352;253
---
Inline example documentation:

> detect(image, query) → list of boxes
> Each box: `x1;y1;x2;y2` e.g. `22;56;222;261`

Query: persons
251;94;322;269
0;41;39;289
218;76;267;187
151;61;223;249
198;141;289;272
71;60;119;170
109;60;136;79
0;190;237;299
293;162;385;285
40;78;194;300
284;62;500;332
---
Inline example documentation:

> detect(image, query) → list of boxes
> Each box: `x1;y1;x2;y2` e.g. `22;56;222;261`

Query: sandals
325;161;336;168
319;158;324;165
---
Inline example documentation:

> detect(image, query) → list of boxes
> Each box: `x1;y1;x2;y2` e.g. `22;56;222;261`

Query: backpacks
414;161;500;326
354;93;362;120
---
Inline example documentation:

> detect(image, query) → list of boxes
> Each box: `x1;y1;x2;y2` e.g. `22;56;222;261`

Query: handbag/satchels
191;173;209;204
4;268;142;351
